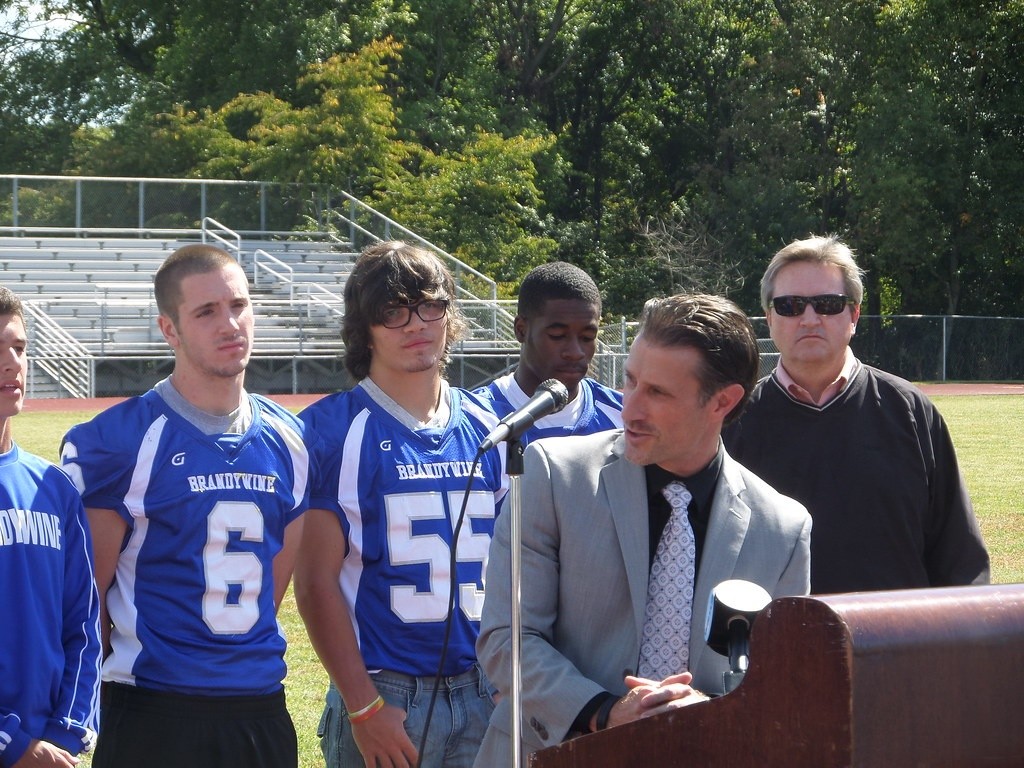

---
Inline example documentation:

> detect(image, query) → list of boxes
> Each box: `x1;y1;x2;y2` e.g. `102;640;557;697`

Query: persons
0;288;103;768
295;241;518;768
60;244;325;768
472;260;624;452
473;292;812;767
721;236;991;594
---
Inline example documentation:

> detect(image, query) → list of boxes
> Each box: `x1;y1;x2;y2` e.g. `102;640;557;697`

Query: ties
637;480;695;687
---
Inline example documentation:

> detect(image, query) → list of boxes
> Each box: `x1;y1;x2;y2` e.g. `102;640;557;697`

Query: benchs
0;236;522;352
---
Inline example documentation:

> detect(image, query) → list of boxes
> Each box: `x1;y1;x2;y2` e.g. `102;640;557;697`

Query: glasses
768;294;853;316
380;298;449;329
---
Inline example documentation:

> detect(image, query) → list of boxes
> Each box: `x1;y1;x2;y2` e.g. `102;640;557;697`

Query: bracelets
596;695;620;731
347;696;384;723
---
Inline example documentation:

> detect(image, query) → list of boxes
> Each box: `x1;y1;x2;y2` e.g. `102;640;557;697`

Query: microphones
703;579;773;695
477;378;569;453
